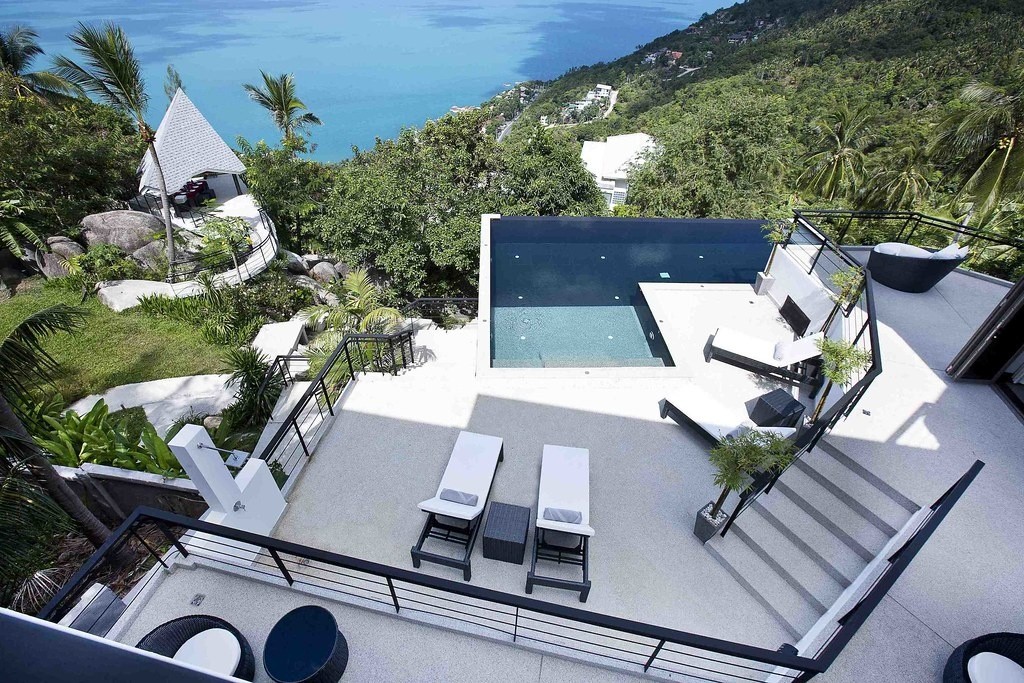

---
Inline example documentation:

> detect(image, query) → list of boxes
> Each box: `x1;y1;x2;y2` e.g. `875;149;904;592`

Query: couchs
134;614;255;682
866;242;970;293
943;632;1024;683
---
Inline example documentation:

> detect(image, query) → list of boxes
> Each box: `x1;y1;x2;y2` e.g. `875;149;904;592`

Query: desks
202;189;216;201
263;605;349;683
749;388;805;428
483;501;531;565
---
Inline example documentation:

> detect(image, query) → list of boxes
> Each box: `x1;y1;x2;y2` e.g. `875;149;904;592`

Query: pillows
439;488;478;507
929;243;960;261
543;507;583;525
773;342;784;362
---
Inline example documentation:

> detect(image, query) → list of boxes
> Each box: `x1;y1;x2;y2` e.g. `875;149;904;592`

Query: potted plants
754;195;800;296
797;337;875;441
809;264;866;339
692;425;801;544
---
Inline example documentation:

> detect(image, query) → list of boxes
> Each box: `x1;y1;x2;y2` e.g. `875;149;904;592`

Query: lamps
198;442;250;470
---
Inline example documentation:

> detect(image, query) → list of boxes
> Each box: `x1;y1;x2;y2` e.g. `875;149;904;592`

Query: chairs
411;430;504;581
705;327;825;400
169;180;209;211
525;443;595;603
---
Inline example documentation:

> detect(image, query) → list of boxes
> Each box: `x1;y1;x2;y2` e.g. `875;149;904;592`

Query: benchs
204;172;218;179
